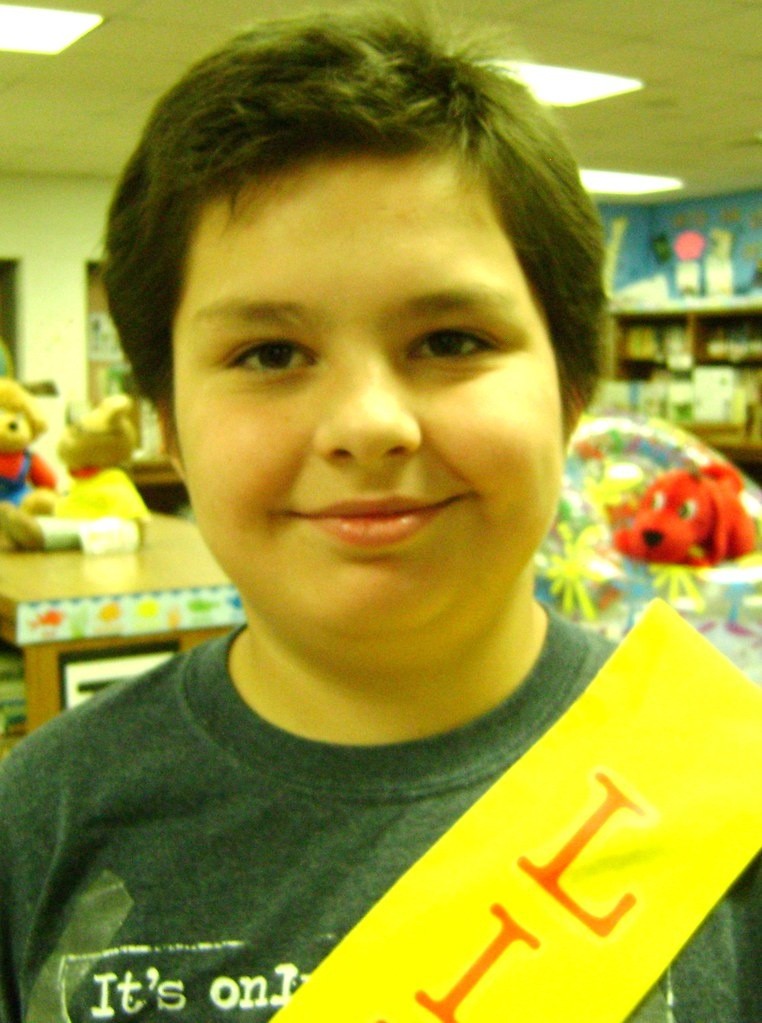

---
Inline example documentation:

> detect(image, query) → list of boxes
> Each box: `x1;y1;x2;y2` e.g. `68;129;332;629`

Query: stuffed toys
0;378;55;506
622;461;755;568
0;396;153;551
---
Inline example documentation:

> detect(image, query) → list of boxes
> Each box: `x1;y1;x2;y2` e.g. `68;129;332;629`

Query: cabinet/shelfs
608;308;762;464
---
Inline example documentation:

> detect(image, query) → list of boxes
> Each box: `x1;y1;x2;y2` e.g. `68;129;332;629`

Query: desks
0;505;245;737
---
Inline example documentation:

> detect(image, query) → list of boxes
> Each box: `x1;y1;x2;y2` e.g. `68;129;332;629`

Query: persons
0;10;762;1023
